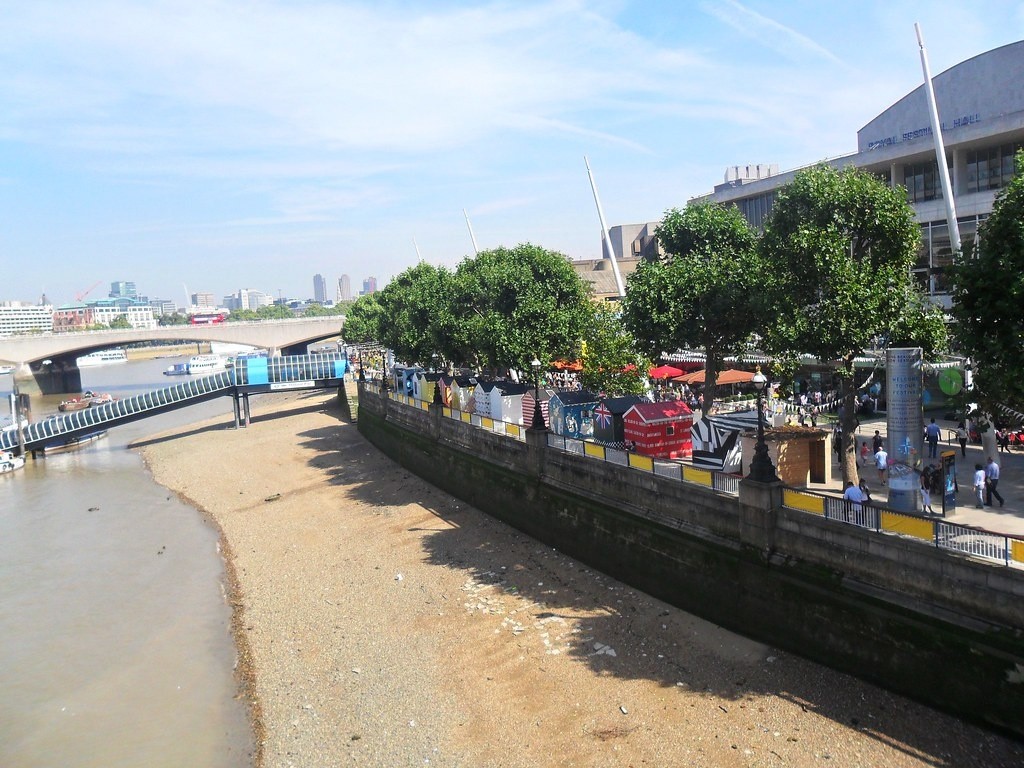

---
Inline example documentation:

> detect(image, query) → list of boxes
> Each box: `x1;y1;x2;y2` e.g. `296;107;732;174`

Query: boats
35;429;107;456
58;391;120;412
163;349;268;376
76;349;129;366
0;451;26;474
311;347;337;354
0;365;16;374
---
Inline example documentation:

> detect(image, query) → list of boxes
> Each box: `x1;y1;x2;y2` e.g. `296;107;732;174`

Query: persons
861;441;870;468
626;440;636;453
833;422;1010;471
735;388;876;427
946;422;969;458
973;464;986;509
926;417;942;459
920;465;936;514
859;477;872;504
872;430;885;467
538;373;704;411
984;456;1005;507
843;481;865;526
873;446;888;486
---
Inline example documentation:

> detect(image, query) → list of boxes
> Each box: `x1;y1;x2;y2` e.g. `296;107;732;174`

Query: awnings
657;350;963;375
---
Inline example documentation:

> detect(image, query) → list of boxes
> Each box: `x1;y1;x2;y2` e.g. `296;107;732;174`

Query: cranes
76;281;101;302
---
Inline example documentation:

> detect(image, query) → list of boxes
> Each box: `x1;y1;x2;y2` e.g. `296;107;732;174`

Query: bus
191;313;224;324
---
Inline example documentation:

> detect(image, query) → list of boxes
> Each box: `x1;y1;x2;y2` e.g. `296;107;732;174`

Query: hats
859;478;865;482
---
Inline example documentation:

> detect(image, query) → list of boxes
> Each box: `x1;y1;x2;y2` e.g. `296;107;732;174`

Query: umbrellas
549;357;766;395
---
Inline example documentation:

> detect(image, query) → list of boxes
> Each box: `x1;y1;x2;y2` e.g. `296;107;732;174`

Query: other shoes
933;456;937;458
928;456;930;458
976;505;983;509
961;454;966;457
930;511;937;514
1000;498;1005;507
923;510;928;514
984;503;992;506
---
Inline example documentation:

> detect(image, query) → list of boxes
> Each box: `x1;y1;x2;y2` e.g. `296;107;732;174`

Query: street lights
357;345;366;380
431;349;443;404
745;365;779;482
531;353;548;430
380;347;389;387
343;342;350;373
